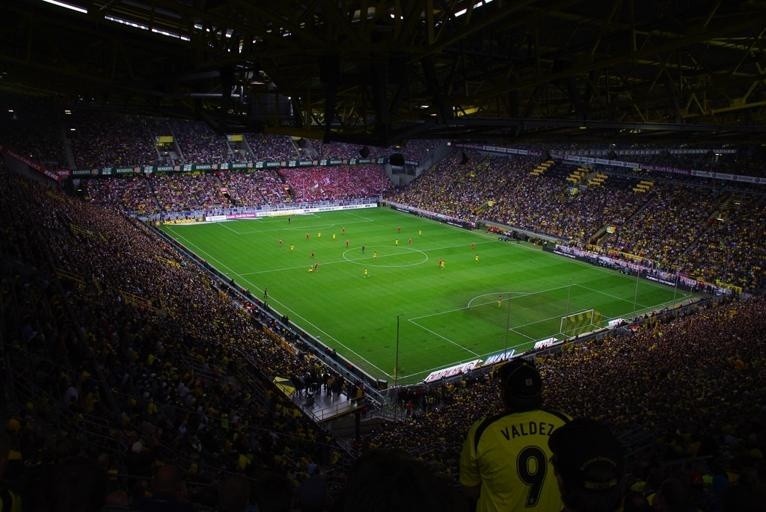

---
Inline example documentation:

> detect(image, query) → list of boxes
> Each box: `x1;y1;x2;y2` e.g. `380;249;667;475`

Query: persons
281;298;765;512
1;149;280;511
275;226;378;279
383;146;766;294
2;107;458;223
